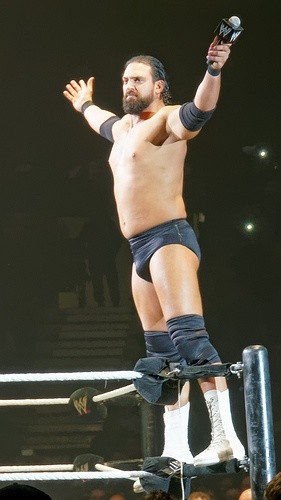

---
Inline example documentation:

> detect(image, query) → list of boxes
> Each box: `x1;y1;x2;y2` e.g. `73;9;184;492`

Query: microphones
207;16;244;64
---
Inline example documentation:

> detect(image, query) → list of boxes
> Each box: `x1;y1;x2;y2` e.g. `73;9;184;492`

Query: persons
0;484;53;500
62;40;246;468
265;472;281;500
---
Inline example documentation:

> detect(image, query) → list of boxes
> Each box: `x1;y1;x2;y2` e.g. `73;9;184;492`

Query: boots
194;388;247;466
132;401;191;494
